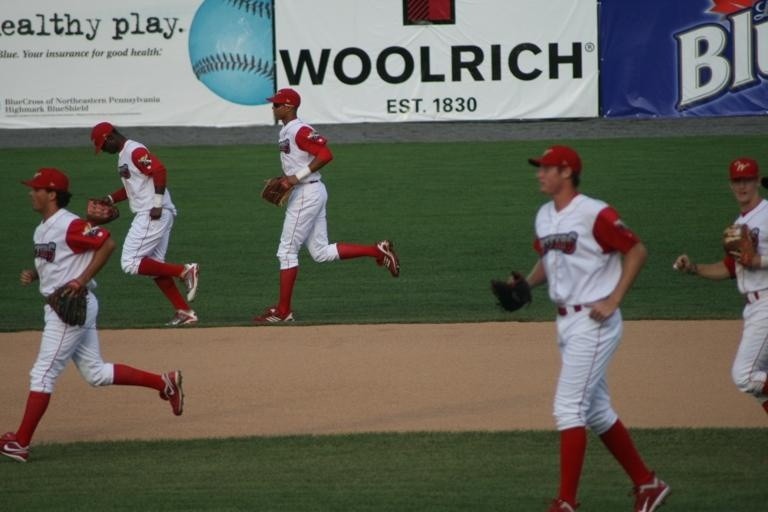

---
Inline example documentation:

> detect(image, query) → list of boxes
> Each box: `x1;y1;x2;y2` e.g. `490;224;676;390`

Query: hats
729;160;759;179
266;89;300;107
528;145;582;174
21;166;68;191
90;123;114;156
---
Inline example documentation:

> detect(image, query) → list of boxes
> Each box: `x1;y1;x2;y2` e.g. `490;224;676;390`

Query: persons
673;157;768;413
252;89;400;322
502;143;672;511
0;167;185;463
90;121;199;328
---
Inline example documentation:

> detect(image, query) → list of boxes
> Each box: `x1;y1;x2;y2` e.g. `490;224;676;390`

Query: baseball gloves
491;270;531;311
47;279;88;326
262;176;295;207
723;223;759;269
86;197;120;223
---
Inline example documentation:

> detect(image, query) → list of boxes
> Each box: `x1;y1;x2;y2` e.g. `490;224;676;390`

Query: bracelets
762;256;765;275
295;165;312;182
152;193;164;208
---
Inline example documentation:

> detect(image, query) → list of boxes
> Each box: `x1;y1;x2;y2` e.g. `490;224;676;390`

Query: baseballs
190;0;275;105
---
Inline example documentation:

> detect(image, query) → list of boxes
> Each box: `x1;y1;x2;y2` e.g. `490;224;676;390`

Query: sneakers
546;500;581;512
160;371;183;416
163;263;200;328
632;476;672;512
0;432;30;463
376;239;400;276
252;307;296;326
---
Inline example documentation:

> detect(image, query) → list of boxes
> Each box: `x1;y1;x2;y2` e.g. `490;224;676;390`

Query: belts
557;305;582;316
